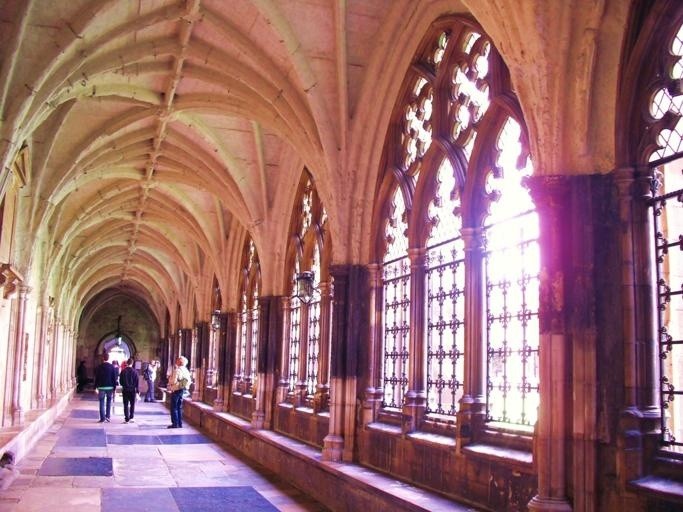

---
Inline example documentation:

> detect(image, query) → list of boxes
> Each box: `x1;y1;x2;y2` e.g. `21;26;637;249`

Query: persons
76;356;128;393
144;359;160;403
92;353;116;423
119;358;140;423
167;355;189;429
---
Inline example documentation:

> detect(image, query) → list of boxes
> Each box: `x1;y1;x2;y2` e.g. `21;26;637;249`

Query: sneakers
168;424;182;428
122;419;134;424
144;399;156;402
98;418;110;423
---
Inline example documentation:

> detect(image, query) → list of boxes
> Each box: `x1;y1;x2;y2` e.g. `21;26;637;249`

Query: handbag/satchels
143;365;149;380
179;378;188;389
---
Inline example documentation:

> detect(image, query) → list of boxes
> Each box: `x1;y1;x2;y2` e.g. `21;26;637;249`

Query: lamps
294;270;321;305
210;309;223;332
115;315;123;346
